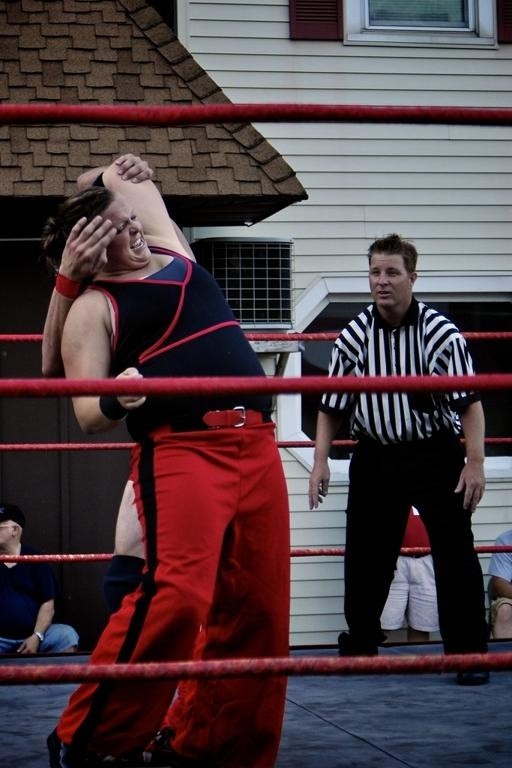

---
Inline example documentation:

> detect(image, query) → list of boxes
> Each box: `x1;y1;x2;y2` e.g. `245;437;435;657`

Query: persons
38;149;200;611
45;156;291;768
487;528;511;640
305;233;492;687
0;503;80;658
380;506;441;643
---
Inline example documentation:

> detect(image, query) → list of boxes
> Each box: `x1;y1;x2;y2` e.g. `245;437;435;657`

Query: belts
200;408;270;431
400;552;431;559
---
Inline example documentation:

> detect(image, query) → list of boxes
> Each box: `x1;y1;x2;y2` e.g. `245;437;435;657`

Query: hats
0;507;31;531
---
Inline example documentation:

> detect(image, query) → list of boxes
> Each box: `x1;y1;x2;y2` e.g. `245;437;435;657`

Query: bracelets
54;272;81;301
98;392;128;422
33;629;45;641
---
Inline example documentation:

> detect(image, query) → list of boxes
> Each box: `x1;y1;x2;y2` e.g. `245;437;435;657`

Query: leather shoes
455;669;492;686
48;729;86;768
